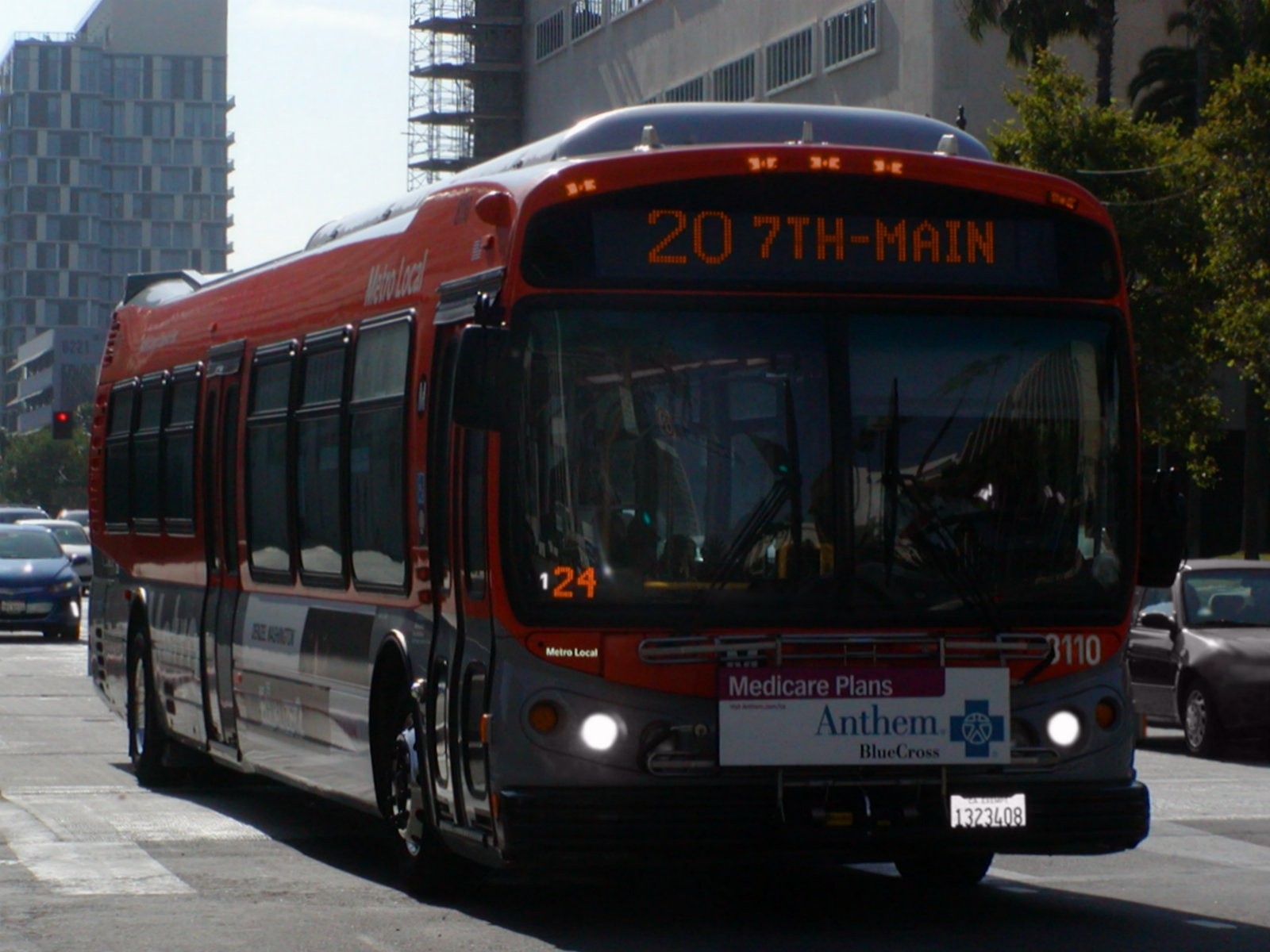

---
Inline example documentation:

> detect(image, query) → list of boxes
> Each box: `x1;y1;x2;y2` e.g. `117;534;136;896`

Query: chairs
1184;584;1201;621
1209;595;1245;620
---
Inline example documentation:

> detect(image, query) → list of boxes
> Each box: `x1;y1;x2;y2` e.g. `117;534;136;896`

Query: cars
0;523;84;642
0;502;92;597
1127;556;1269;760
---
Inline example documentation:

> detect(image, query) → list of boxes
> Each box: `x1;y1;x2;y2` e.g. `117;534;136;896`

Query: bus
86;101;1151;908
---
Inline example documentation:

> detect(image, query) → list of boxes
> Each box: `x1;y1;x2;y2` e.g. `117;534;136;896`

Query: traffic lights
54;410;74;439
